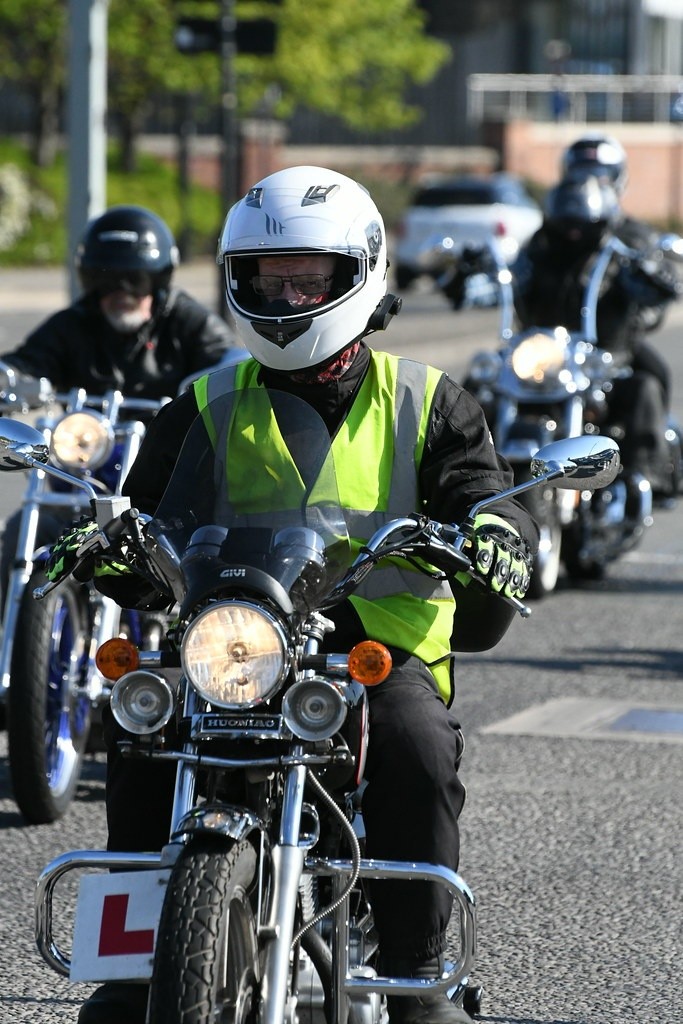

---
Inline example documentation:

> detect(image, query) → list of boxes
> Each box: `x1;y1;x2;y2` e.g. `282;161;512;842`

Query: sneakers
77;979;150;1023
390;951;477;1024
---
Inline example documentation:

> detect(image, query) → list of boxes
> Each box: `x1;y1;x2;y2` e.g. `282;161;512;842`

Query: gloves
452;512;534;599
43;514;135;584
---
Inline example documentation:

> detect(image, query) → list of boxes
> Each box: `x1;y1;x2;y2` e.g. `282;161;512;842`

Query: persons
79;164;542;1023
542;137;683;505
514;170;671;518
0;201;253;724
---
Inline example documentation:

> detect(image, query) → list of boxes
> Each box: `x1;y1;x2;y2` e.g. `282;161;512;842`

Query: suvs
395;171;546;291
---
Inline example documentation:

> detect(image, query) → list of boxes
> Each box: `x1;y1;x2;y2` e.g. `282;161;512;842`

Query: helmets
562;134;629;196
75;205;179;288
214;165;403;371
541;171;623;250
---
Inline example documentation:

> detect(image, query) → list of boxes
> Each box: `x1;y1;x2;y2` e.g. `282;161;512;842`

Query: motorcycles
460;226;678;601
0;364;180;820
1;407;622;1024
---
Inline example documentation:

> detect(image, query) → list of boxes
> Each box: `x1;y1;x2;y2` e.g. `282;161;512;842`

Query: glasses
248;272;336;298
89;268;160;297
